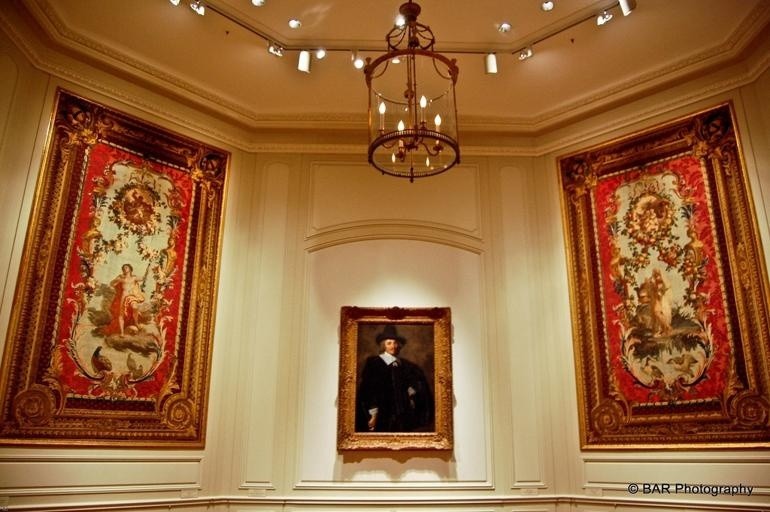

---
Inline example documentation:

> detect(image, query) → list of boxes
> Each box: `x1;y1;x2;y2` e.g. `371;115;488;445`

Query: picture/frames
556;99;770;449
339;305;454;450
1;84;232;451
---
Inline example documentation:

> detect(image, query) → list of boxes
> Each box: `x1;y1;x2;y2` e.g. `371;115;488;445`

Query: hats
375;325;407;346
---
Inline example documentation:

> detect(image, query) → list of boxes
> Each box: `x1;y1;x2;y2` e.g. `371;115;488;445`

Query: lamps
291;44;512;79
363;2;461;182
510;0;638;67
170;0;290;62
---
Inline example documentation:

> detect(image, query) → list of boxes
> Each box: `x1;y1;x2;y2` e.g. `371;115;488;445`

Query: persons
111;264;146;337
355;323;435;433
